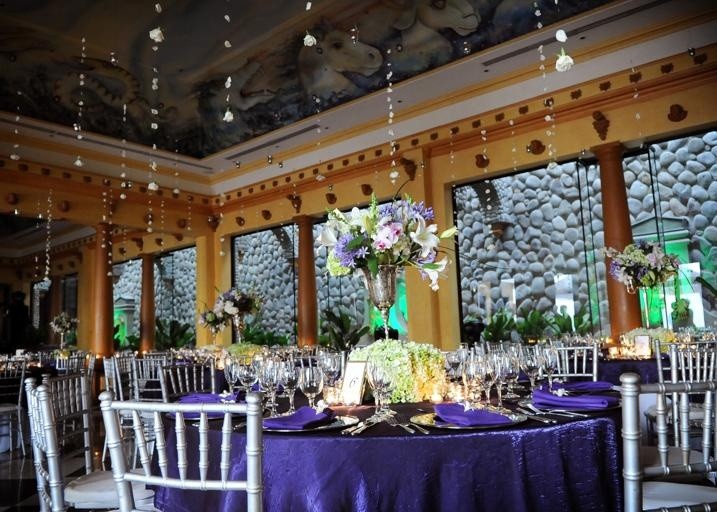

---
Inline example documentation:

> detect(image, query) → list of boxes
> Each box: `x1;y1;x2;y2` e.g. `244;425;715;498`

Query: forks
515;401;591;424
385;413;431;434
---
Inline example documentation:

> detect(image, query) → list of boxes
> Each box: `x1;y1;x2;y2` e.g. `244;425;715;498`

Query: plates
515;395;622;412
163;407;251;421
408;409;529;429
260;411;359;432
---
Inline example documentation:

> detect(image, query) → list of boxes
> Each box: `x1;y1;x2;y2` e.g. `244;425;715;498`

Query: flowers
198;284;265;334
316;178;513;293
49;312;80;335
600;239;695;313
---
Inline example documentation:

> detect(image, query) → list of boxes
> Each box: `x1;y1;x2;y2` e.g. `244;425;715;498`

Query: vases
361;264;399;341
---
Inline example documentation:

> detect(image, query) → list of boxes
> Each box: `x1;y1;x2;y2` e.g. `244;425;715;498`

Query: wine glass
167;346;218;367
365;354;394;420
217;340;346;419
437;337;557;408
556;326;717;358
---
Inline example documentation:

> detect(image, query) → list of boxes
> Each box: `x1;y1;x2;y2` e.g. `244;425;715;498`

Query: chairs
0;326;716;512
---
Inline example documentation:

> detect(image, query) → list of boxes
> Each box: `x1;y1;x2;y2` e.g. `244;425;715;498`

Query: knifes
340;413;380;436
